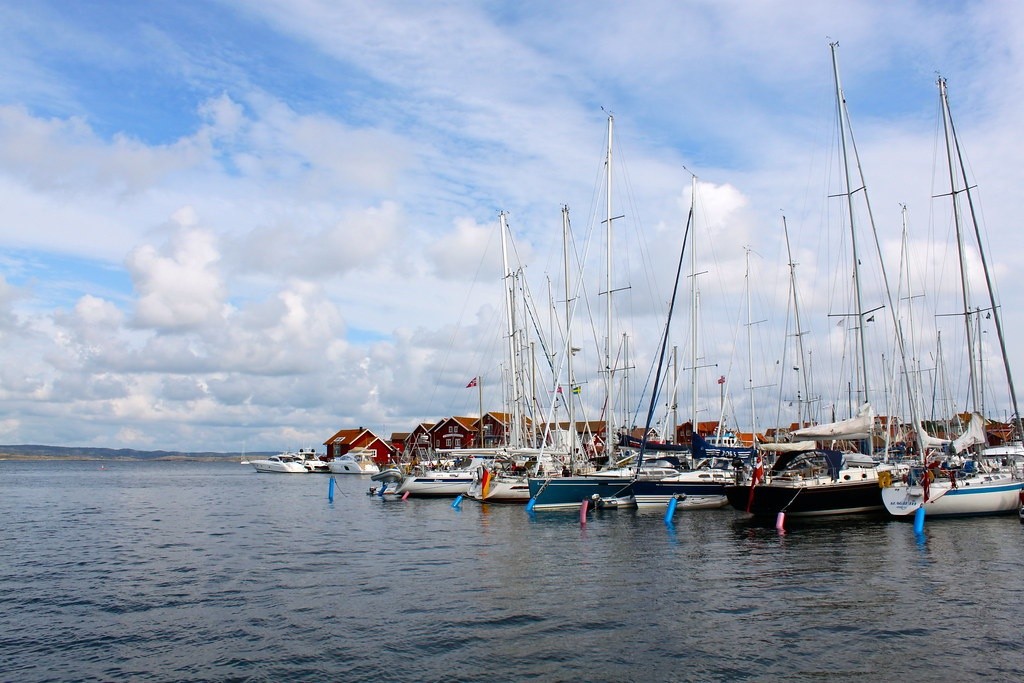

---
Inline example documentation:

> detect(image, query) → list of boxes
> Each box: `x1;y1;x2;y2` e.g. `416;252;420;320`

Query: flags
744;453;764;515
571;386;582;395
466;377;478;388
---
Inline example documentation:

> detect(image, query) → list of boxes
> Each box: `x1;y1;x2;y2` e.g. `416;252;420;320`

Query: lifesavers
878;472;891;489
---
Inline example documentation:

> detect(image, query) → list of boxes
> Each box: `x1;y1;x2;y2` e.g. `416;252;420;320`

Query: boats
249;450;308;473
292;446;330;472
326;446;380;475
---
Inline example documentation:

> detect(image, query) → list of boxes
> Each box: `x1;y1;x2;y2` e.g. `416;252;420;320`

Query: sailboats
241;440;250;464
366;38;1024;522
